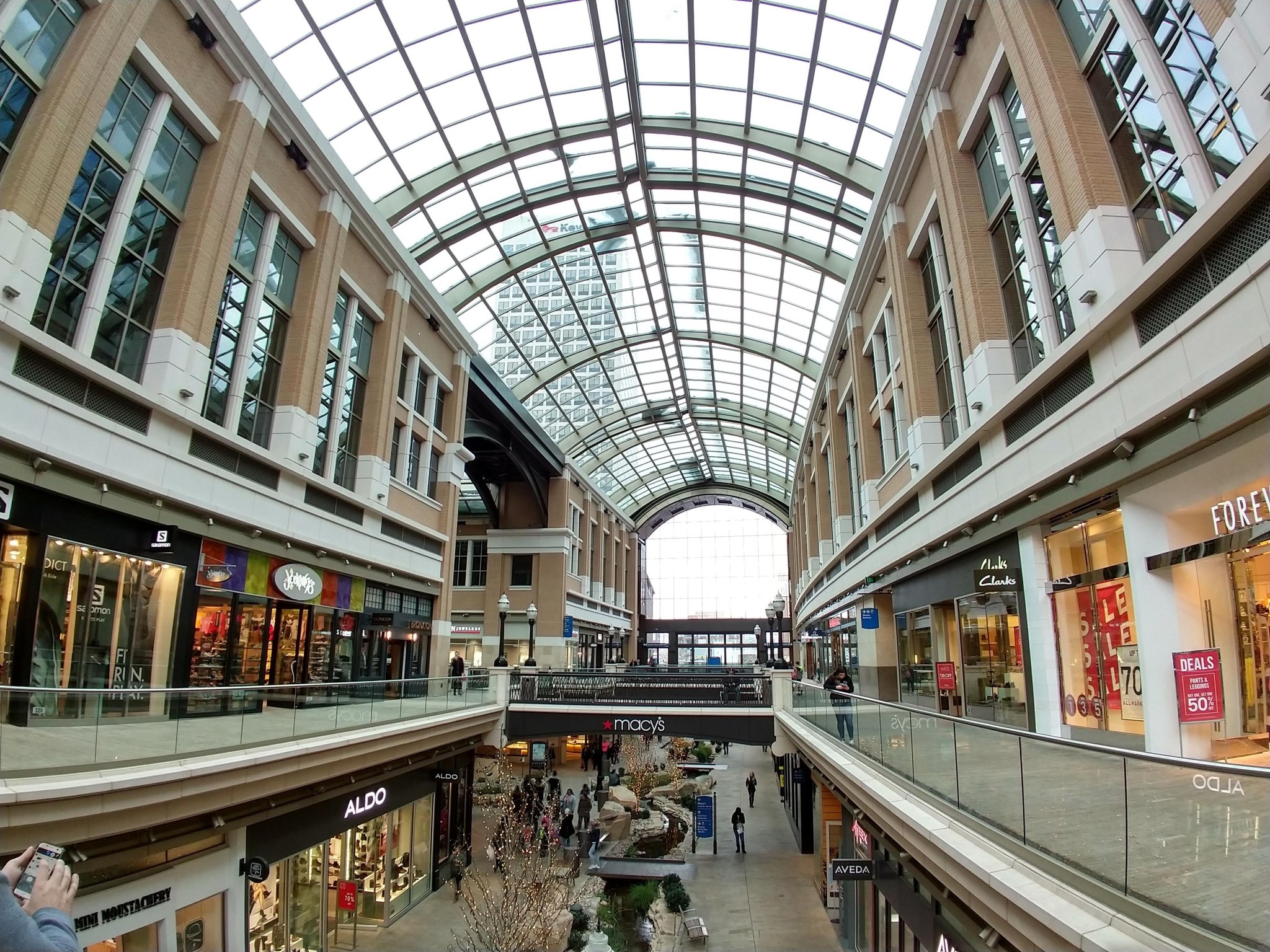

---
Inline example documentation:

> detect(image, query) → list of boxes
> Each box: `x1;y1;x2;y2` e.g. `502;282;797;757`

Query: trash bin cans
762;680;773;704
578;829;592;858
563;846;580;878
598;790;609;812
522;677;535;701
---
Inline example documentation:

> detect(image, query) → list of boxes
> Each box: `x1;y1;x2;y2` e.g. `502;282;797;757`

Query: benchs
545;860;578;890
595;828;611;852
672;903;712;948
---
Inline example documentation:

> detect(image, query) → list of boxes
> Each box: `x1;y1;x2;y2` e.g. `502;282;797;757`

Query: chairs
978;676;1022;711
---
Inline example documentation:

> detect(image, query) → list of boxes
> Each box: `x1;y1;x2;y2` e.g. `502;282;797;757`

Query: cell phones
12;842;66;900
840;684;845;687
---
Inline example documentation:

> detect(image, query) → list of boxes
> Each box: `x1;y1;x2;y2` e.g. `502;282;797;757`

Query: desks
1003;672;1026;706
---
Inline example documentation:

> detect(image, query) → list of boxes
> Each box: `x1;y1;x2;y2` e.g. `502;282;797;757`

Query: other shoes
736;849;740;853
849;739;853;745
841;739;846;743
588;864;600;870
250;885;309;952
292;819;424;897
742;850;747;854
492;865;496;873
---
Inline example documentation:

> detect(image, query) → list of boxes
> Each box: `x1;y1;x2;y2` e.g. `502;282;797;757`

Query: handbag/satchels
737;823;744;834
487;844;496;861
580;760;584;769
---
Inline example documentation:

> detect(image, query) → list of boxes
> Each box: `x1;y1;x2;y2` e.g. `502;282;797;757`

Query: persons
547;743;556;772
746;771;757;808
762;745;767;752
640;734;653;751
754;658;762;672
447;845;467;904
823;666;854;746
609;768;621;786
607;657;637;666
588;822;602;869
789;661;803;696
657;735;663;743
450;651;464;696
0;846;81;952
731;807;747;854
722;741;729;755
722;668;741;704
815;657;821;683
647;656;657;667
490;770;594;880
581;738;620;772
903;659;916;697
250;882;272;918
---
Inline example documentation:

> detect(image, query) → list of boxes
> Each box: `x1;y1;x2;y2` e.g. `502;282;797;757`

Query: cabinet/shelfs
977;624;1002;662
248;809;430;952
184;630;353;713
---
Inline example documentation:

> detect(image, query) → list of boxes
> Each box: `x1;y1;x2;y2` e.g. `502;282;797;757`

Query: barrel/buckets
563;846;580;878
578;829;592;858
597;789;609;812
602;759;610;778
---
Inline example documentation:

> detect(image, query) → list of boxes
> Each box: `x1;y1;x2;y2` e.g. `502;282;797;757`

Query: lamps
179;388;194;399
1078;290;1097;305
411;631;420;645
794;403;1210;633
2;285;21;300
30;453;432;589
383;628;393;642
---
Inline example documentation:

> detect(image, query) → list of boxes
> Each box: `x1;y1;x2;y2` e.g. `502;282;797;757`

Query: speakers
251;529;262;538
33;457;51;472
1112;441;1133;461
959;525;974;537
921;547;929;555
316;549;327;557
